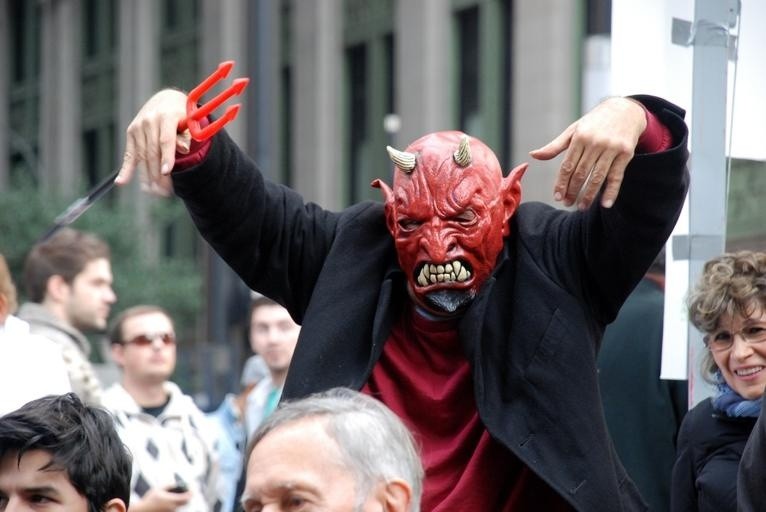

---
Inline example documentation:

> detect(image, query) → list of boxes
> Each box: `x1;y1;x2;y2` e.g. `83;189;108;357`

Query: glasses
703;322;766;351
121;333;175;345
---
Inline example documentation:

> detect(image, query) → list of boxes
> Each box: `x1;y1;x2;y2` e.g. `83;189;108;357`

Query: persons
594;247;686;499
115;89;691;512
96;304;224;512
2;229;116;412
670;249;764;510
242;292;302;507
234;385;424;512
0;391;134;511
0;253;17;330
208;355;272;511
